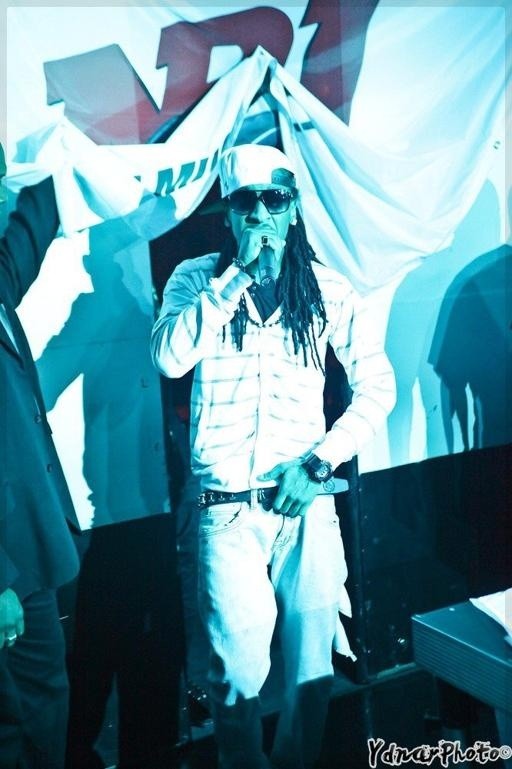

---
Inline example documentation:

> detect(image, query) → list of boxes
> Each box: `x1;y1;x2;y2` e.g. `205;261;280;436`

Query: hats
217;143;300;199
0;142;7;177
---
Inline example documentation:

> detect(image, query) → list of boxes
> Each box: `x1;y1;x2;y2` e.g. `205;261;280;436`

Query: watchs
301;450;334;483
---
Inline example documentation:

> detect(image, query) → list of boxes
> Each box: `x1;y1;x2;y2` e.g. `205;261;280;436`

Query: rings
6;634;17;642
262;236;270;246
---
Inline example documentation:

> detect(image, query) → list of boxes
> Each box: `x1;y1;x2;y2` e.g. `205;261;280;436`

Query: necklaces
248;312;284;329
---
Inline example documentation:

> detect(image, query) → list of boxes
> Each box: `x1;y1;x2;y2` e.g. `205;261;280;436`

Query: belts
196;478;349;511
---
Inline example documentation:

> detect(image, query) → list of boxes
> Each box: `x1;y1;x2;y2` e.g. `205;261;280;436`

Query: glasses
222;189;294;215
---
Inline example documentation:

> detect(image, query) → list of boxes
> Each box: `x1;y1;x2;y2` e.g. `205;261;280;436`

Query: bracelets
232;255;247;274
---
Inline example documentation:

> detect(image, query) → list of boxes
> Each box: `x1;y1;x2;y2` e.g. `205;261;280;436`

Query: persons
148;145;398;768
0;142;82;768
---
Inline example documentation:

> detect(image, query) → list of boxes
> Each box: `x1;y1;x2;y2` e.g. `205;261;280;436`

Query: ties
0;303;20;355
